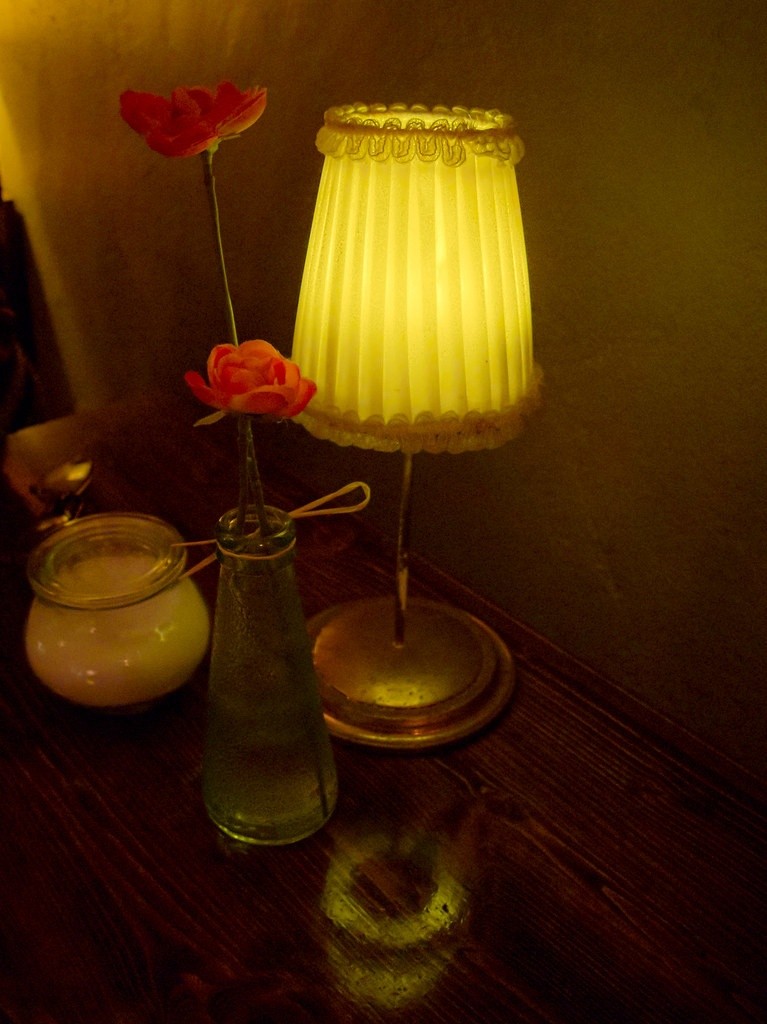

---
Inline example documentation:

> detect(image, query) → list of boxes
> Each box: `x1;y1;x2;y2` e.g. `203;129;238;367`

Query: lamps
289;102;544;754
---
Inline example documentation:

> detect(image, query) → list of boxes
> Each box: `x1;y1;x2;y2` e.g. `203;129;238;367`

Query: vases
199;508;337;846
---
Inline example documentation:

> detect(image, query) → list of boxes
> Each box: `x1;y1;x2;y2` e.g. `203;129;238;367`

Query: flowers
122;80;337;534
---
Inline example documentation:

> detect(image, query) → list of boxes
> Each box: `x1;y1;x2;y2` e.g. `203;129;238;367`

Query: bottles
22;510;210;708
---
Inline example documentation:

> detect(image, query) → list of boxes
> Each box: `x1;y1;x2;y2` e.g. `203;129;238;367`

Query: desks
1;396;767;1024
